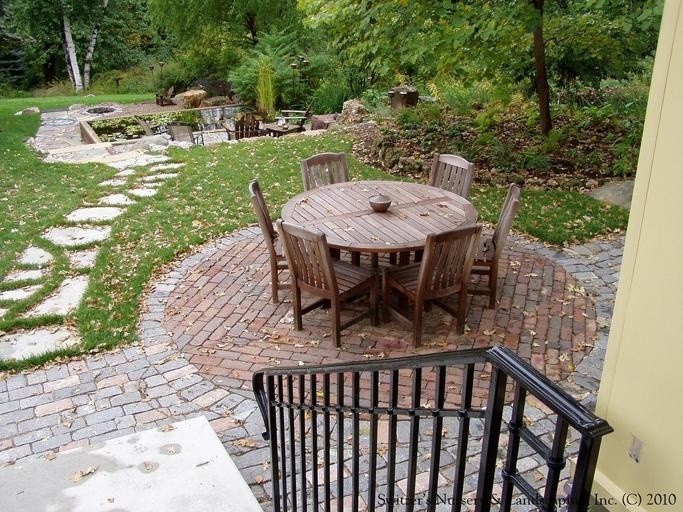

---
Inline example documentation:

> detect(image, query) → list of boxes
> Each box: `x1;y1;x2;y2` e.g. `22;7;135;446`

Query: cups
277;117;285;127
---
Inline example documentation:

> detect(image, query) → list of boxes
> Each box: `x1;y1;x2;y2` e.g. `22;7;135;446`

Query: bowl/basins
368;194;392;213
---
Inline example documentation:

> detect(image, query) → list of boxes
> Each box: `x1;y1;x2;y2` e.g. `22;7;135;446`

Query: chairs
133;115;204;145
221;98;315;141
155;86;174;106
